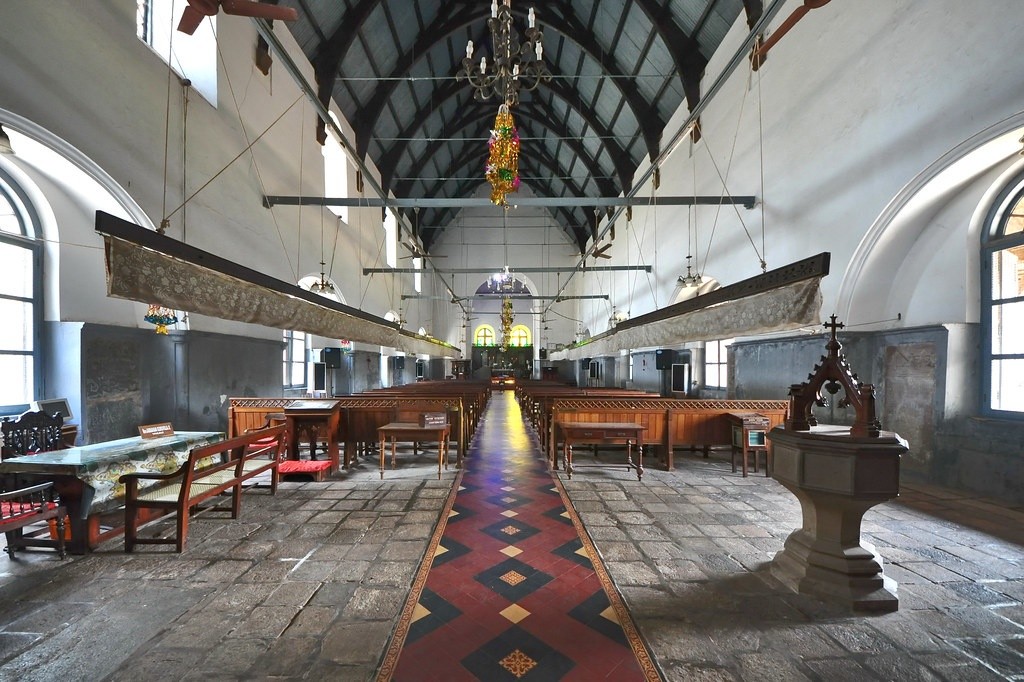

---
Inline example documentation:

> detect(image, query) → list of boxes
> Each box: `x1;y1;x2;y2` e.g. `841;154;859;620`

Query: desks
377;423;451;480
562;421;647;483
0;431;227;555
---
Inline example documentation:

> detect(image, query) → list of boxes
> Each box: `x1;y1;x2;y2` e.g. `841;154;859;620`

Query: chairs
0;483;71;562
3;412;64;451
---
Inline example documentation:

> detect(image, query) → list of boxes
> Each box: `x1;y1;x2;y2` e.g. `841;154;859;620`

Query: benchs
118;424;285;554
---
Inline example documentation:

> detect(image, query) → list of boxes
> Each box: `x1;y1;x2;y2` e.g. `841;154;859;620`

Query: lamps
459;0;543;212
563;117;730;344
144;0;181;337
307;205;442;340
486;265;528;292
499;294;514;348
